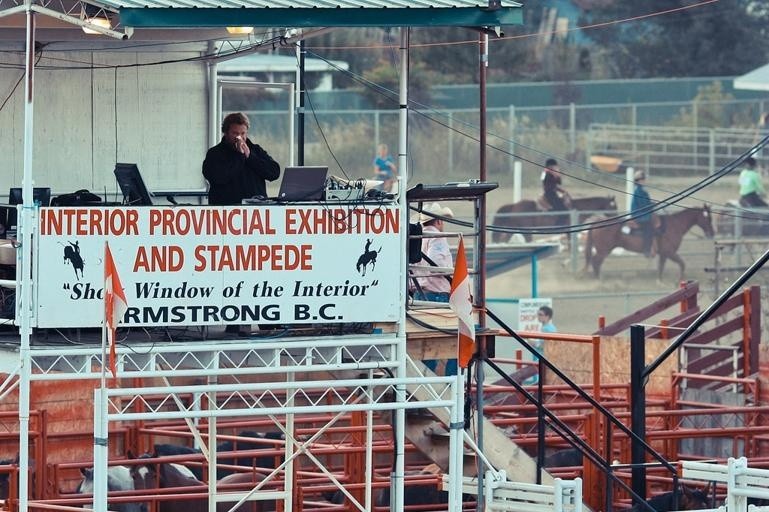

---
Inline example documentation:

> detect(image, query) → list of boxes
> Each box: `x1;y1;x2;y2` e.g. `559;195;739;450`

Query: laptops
260;166;328;201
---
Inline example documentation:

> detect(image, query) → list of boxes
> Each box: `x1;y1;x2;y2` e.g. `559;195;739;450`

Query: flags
447;231;477;371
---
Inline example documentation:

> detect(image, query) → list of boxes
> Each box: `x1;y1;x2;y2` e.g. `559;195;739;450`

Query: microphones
167;195;177;204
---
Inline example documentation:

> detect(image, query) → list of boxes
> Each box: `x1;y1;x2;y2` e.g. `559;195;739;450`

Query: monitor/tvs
114;163;153;206
8;188;50;231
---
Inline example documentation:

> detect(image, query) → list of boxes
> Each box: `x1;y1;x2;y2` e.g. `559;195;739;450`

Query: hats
409;203;454;225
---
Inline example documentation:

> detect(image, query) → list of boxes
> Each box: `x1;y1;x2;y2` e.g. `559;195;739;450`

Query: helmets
545;160;557;165
634;170;645;180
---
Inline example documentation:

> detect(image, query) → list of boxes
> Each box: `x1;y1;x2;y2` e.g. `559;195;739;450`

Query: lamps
81;4;112;35
226;26;254;35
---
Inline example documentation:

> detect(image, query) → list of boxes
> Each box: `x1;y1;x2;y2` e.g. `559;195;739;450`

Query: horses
378;449;583;512
492;195;620;253
126;450;276;512
622;482;712;511
79;462;200;512
715;200;769;264
576;202;715;288
151;430;287;483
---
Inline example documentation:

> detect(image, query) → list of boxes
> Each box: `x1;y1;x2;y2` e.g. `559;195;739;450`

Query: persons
750;112;769;179
371;142;398;193
525;305;562;387
629;169;661;261
538;158;572;230
200;108;292;335
737;156;768;237
407;202;469;418
